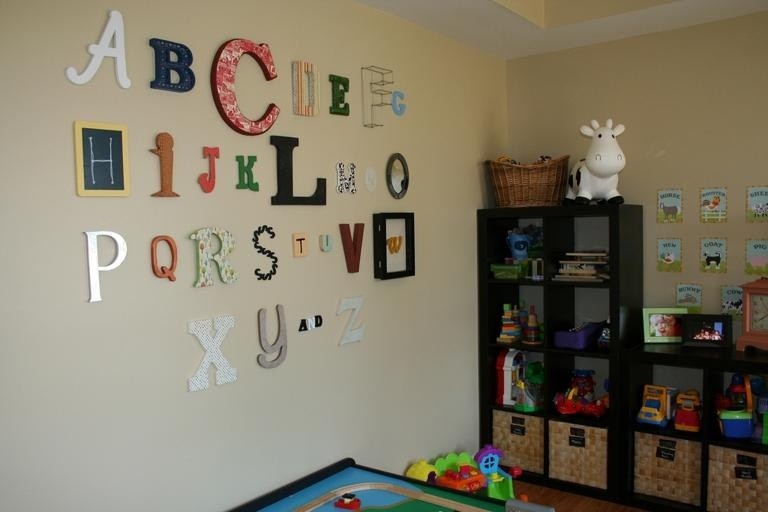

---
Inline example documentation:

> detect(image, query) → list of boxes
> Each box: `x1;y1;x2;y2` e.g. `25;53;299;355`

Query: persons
693;329;722;340
650;313;682;337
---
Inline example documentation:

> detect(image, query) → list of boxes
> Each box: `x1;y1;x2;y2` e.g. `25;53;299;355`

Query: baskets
485;154;570;208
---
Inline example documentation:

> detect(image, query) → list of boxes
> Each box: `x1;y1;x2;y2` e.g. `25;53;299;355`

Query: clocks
736;276;768;352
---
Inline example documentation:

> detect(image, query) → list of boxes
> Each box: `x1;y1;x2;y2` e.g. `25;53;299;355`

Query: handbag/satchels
552;323;601;349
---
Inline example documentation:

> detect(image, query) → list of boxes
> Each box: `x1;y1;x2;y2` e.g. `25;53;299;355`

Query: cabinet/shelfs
477;203;644;507
617;342;767;512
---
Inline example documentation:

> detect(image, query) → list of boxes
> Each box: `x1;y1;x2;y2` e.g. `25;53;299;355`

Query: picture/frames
373;212;415;280
642;307;688;344
74;121;130;197
683;314;733;351
